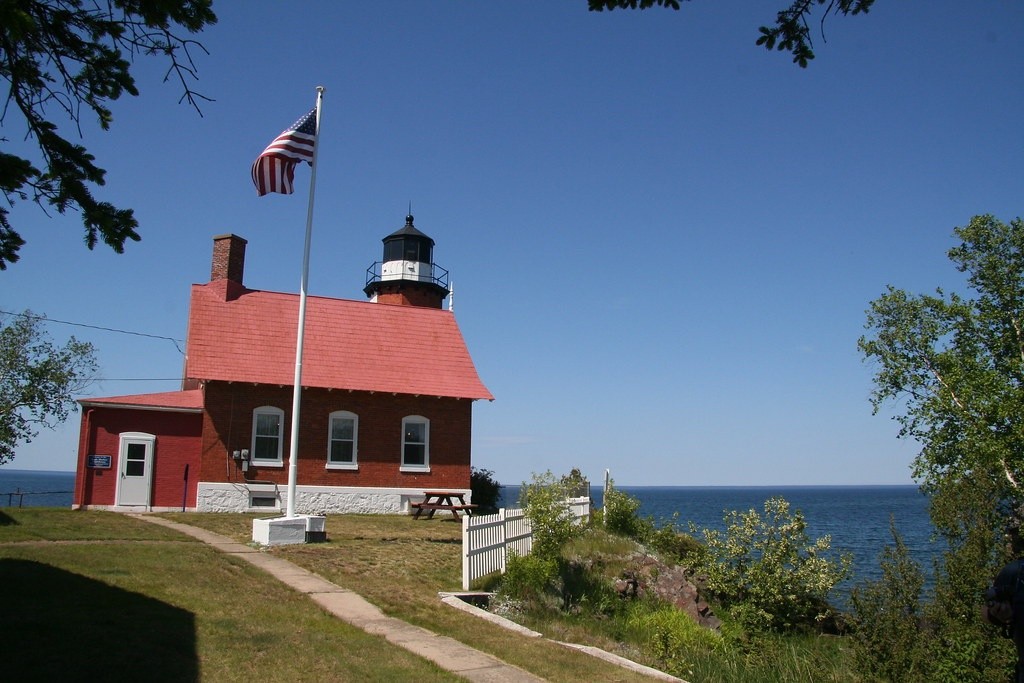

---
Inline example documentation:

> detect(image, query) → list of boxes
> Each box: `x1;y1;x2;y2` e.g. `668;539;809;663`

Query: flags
251;100;317;197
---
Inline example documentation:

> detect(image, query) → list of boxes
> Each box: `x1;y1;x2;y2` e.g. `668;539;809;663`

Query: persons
982;557;1024;683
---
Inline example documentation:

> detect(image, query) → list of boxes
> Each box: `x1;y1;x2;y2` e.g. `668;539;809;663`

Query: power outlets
241;449;248;459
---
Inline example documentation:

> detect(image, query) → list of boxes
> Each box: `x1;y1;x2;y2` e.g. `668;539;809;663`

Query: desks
412;491;471;520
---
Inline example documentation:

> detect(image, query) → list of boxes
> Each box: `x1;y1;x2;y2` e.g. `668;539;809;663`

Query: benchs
411;502;479;521
